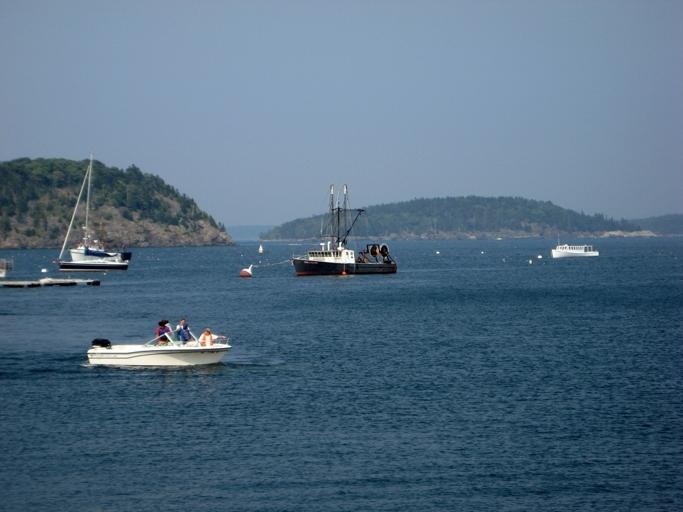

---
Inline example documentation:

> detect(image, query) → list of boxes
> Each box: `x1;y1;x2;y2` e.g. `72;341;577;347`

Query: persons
175;318;192;342
161;319;173;335
196;327;226;346
155;319;170;344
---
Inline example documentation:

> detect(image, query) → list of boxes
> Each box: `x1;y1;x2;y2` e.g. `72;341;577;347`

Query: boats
84;341;235;369
289;180;399;278
548;244;603;259
238;264;254;277
258;243;264;253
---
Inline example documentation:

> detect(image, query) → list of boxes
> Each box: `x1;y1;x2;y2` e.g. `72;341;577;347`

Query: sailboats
56;164;131;273
74;151;134;262
